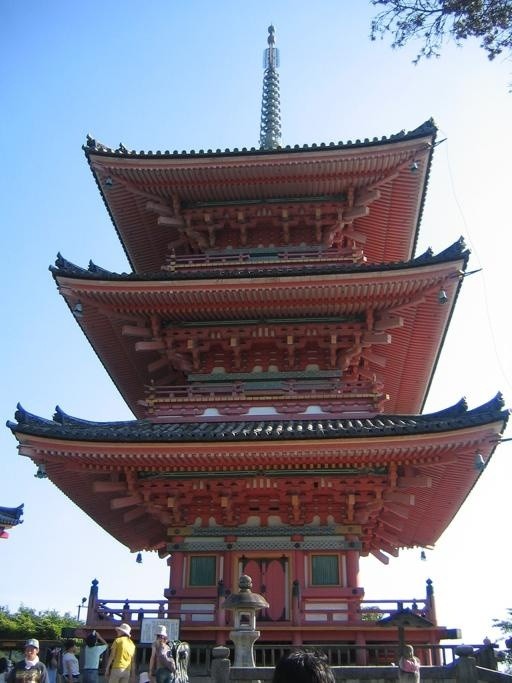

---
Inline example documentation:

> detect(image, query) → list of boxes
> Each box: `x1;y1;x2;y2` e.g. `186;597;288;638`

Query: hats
139;671;150;683
116;624;132;637
25;638;39;649
155;625;166;635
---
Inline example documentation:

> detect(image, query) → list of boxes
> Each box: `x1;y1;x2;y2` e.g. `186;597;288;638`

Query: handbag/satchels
159;642;176;671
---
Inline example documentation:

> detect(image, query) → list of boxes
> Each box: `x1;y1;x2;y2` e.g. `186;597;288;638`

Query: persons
62;638;81;682
105;622;137;682
149;624;176;682
44;645;62;682
9;637;51;682
397;643;422;682
140;671;152;682
271;646;336;681
0;656;11;682
84;630;110;682
